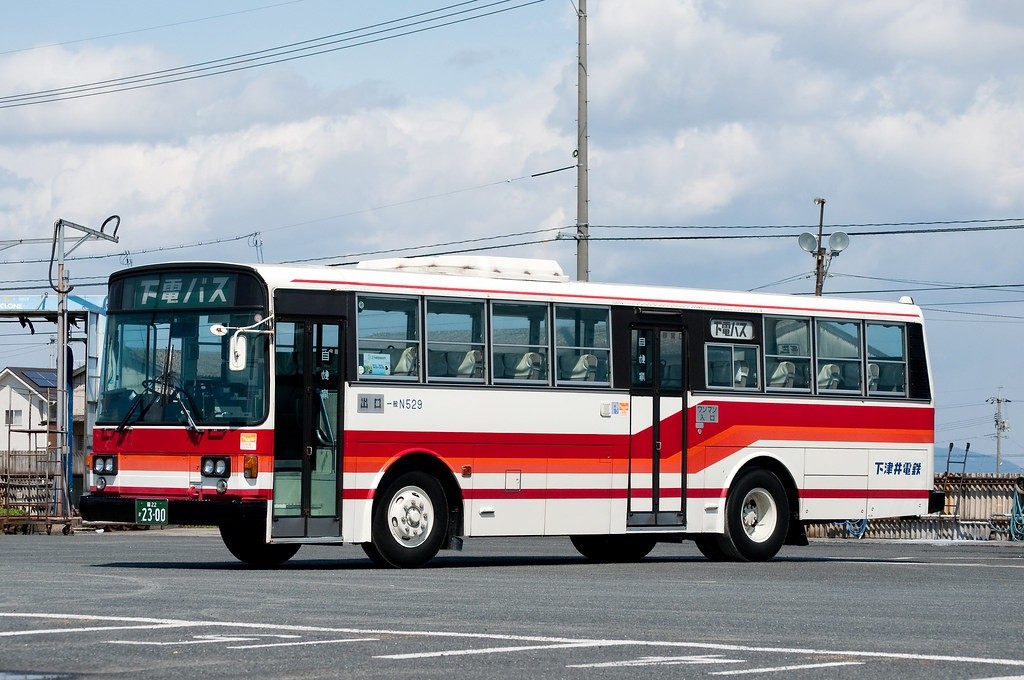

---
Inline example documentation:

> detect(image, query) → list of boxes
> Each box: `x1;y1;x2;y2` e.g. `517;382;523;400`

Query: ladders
940;441;971;515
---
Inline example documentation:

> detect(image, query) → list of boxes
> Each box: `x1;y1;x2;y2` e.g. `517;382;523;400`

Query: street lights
799;196;852;296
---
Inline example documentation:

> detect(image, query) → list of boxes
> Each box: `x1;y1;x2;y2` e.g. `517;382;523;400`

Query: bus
76;253;939;570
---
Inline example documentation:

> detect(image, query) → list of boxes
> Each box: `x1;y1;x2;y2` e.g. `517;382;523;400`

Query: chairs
380;344;903;393
